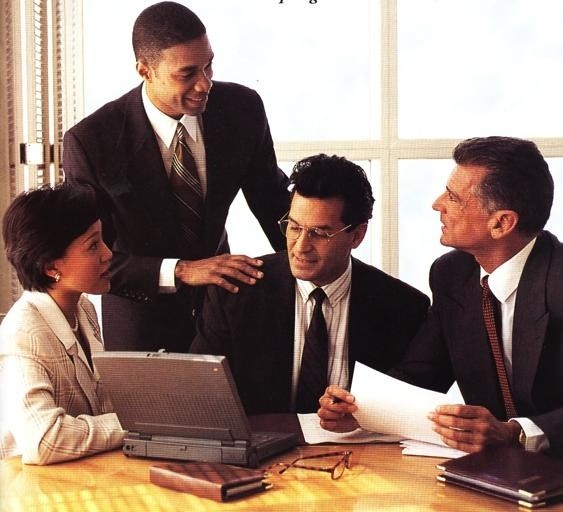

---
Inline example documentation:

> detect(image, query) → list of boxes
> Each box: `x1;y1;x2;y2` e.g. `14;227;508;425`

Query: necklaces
70;311;79;332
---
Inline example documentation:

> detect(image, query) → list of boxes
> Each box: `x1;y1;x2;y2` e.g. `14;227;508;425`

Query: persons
62;2;294;352
317;135;563;455
188;152;431;416
0;183;129;466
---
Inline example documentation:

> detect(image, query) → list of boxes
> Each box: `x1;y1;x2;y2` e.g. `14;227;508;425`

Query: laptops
90;351;298;466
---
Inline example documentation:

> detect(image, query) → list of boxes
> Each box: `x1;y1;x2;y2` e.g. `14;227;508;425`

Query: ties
294;289;330;414
482;279;518;421
168;125;206;225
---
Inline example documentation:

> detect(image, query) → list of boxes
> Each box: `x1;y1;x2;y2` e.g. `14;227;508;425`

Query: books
149;460;273;503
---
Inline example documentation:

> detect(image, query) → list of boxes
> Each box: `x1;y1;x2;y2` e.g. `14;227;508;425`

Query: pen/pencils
329;396;342;405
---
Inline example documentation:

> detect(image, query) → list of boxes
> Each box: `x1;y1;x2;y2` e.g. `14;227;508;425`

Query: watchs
518;427;526;446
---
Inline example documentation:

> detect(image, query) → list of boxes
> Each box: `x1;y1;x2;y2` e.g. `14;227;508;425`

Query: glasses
263;448;355;481
277;211;353;247
